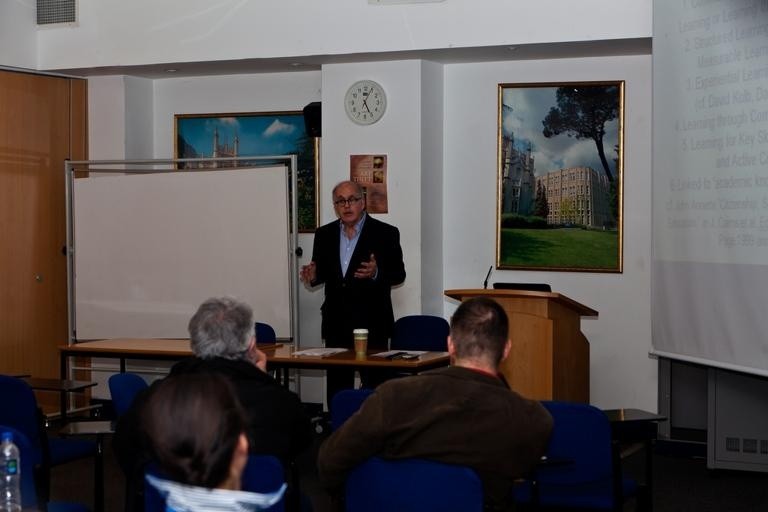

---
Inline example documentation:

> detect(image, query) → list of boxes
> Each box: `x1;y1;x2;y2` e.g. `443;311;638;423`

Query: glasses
332;197;362;207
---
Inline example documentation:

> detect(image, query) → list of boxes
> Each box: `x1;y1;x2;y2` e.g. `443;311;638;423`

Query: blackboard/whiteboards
73;164;293;342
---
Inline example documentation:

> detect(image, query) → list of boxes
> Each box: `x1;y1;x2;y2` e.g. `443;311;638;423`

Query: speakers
303;101;321;137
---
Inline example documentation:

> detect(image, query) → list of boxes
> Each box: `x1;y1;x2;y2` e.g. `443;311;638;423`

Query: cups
353;328;369;355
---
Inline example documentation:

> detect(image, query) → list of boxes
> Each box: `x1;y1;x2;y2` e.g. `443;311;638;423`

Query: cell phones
401;354;420;359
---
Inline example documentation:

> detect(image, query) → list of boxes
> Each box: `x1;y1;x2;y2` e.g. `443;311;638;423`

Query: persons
117;298;311;511
311;296;554;511
301;182;405;410
134;370;288;512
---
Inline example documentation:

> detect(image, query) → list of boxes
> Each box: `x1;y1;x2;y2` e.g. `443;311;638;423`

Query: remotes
386;352;407;361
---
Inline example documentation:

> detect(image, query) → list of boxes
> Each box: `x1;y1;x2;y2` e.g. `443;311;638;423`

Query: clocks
345;80;387;125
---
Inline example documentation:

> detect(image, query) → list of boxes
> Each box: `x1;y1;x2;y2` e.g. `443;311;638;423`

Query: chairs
0;425;87;511
389;316;450;352
108;374;149;418
142;454;287;511
327;389;375;432
344;462;484;512
515;399;637;512
255;323;275;374
0;373;98;462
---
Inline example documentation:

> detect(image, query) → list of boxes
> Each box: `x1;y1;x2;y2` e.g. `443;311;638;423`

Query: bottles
0;432;21;512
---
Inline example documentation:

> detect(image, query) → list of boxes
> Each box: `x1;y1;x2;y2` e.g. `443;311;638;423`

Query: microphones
484;265;492;289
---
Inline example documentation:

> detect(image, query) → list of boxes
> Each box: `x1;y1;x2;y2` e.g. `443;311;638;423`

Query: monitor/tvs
494;281;552;292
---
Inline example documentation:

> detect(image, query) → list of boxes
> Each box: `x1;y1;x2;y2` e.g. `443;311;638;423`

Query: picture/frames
494;80;626;273
174;110;320;235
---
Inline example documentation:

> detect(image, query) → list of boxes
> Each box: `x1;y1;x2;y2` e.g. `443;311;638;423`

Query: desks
257;345;449;391
604;408;668;509
57;421;114;511
17;376;97;427
60;339;281;425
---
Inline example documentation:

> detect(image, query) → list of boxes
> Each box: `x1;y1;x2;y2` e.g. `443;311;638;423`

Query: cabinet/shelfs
703;364;767;470
